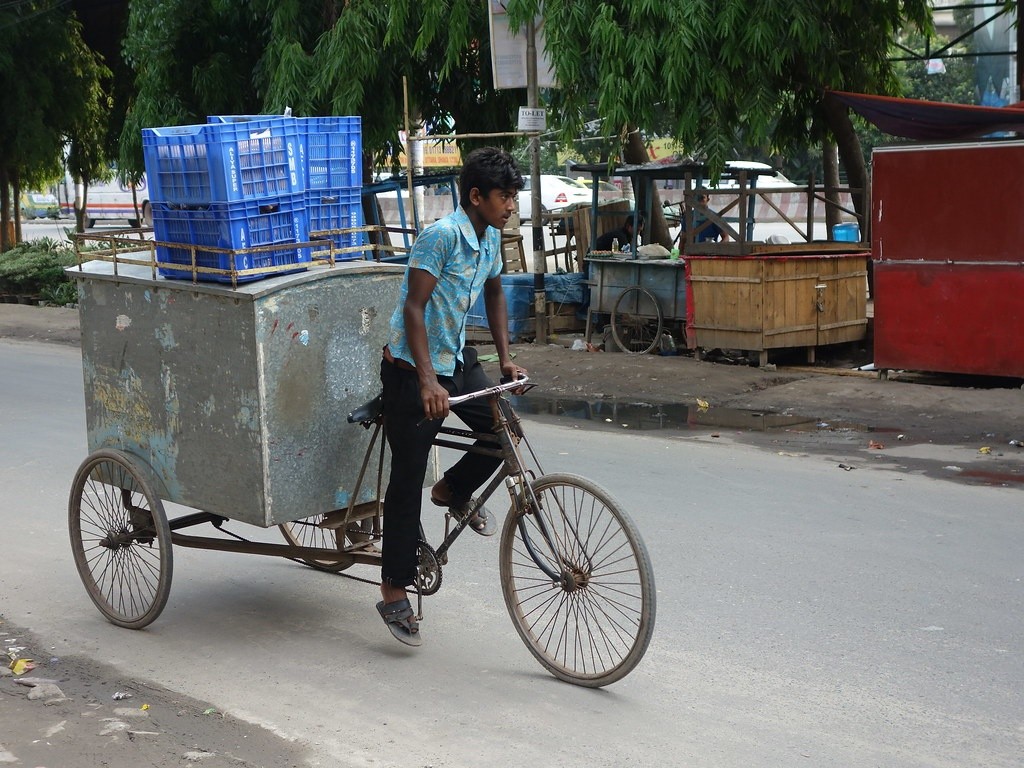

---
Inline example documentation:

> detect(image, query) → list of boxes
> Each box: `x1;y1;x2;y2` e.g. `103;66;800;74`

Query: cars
20;192;59;219
516;173;595;226
574;178;624;202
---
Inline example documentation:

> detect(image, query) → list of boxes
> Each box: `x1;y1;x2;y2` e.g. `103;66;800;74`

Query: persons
438;183;441;189
582;214;646;324
376;147;528;646
424;185;434;196
679;186;729;255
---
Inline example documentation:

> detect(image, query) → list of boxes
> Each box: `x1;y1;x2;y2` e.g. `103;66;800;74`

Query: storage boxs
141;113;364;284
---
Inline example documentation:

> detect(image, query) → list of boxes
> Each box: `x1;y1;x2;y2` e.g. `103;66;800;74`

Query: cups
670;249;680;260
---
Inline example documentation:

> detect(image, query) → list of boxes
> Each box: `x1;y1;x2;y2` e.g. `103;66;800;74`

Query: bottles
611;238;619;253
637;235;642;245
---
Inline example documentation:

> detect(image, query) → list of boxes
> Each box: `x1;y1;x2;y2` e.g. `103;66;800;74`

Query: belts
384;346;416;371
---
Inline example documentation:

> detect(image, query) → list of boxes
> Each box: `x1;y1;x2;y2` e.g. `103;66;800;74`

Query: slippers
376;598;423;646
431;489;498;536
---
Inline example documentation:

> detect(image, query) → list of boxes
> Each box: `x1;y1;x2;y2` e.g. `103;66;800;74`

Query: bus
59;162;159;228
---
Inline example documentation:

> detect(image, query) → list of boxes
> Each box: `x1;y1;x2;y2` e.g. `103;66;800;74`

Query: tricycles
59;225;657;688
